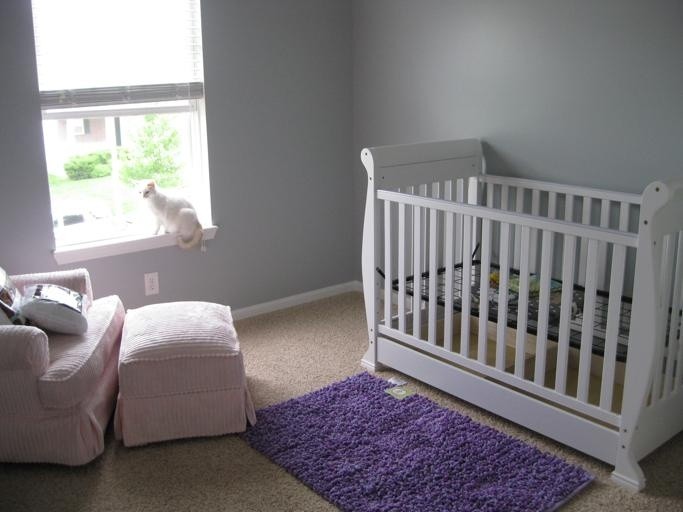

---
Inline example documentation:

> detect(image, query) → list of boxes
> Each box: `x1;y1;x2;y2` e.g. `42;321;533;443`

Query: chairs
0;262;123;470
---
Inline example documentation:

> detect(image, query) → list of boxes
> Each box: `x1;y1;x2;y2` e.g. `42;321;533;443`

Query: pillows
0;266;29;325
19;283;90;335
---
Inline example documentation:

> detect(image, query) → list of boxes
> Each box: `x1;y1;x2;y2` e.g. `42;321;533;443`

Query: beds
353;138;681;494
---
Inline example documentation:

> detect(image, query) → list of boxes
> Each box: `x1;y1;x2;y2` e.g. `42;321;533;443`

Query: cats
137;182;204;250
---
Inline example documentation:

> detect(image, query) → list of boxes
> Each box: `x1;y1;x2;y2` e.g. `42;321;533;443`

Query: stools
114;299;257;450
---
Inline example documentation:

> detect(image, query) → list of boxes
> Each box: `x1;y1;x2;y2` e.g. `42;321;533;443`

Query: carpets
232;366;599;511
